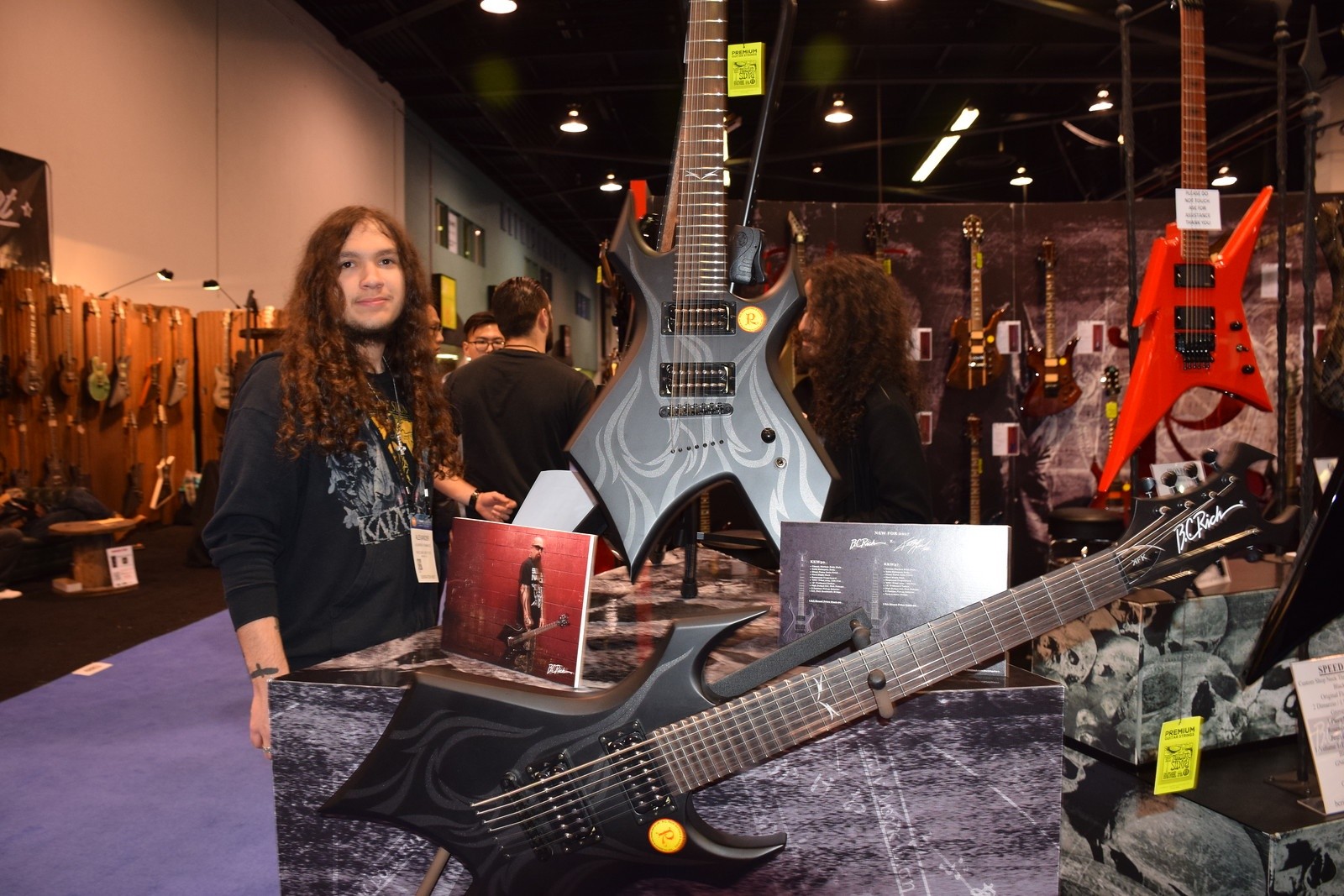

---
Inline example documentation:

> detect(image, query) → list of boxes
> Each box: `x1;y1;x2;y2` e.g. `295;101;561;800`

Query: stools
1044;506;1123;572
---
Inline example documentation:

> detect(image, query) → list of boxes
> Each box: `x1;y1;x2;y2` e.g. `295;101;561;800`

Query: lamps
1211;160;1237;186
100;269;173;297
824;93;853;123
599;169;622;191
203;279;240;309
1089;85;1113;111
559;103;588;133
910;99;979;182
1009;164;1033;186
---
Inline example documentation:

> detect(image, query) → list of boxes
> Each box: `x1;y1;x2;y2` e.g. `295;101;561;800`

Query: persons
425;303;445;358
461;311;505;360
794;253;934;523
497;537;544;669
0;527;24;600
201;205;518;761
442;276;596;525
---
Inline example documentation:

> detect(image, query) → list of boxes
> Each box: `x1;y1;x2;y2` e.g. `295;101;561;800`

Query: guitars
211;310;236;411
1025;235;1083;418
1235;452;1343;689
3;285;190;518
1090;365;1131;512
296;443;1279;896
1093;1;1276;494
558;1;844;588
942;213;1011;390
496;613;571;664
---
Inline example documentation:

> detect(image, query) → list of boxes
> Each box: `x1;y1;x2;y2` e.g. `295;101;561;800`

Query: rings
262;745;271;754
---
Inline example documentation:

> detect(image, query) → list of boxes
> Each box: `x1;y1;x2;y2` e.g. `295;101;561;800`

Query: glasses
532;545;544;552
468;340;506;352
430;326;443;336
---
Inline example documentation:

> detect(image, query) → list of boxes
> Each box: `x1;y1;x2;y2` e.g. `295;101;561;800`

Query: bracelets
469;488;482;509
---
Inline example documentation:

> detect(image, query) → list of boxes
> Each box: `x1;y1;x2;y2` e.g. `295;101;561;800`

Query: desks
49;517;139;598
268;543;1342;896
